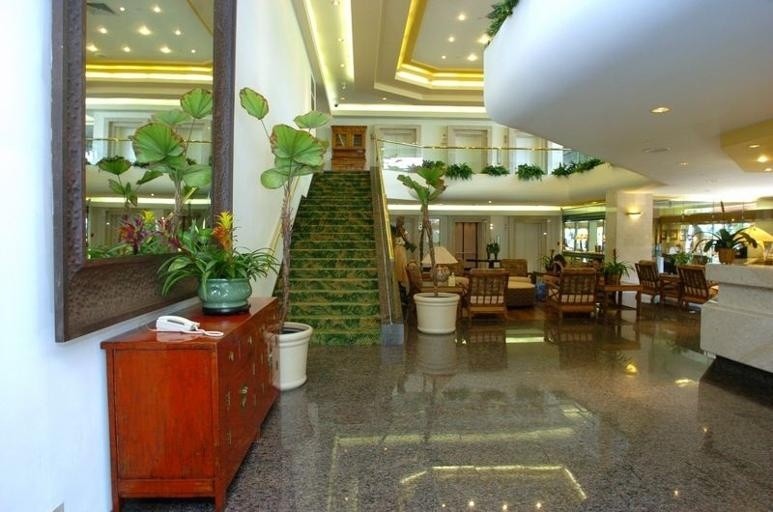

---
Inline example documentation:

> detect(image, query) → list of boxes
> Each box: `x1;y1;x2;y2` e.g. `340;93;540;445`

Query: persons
589;260;606;285
552;254;567;278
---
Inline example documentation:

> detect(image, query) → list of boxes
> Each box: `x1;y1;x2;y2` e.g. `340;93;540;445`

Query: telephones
156;316;200;332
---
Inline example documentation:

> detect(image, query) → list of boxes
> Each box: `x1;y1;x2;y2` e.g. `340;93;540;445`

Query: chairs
499;257;535;305
405;255;510;330
636;259;666;304
544;316;598;370
659;272;681;303
461;320;508;371
678;265;720;313
542;262;600;323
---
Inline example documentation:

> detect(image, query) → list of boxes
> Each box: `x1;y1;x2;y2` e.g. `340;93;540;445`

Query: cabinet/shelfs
96;294;283;511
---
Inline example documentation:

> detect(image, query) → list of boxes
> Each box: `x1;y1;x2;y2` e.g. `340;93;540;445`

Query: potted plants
410;336;463;472
485;243;492;259
604;259;634;282
538;248;566;275
692;225;759;266
396;163;461;335
492;241;499;260
156;211;284;315
239;86;329;392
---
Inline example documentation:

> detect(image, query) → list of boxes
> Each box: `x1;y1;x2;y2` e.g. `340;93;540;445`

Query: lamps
420;245;458;282
738;226;771;259
575;234;587;250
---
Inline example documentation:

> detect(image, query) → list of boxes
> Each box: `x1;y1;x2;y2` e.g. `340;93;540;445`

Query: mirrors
49;0;237;344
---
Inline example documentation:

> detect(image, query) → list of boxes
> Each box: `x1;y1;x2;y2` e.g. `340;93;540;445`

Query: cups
763;241;773;260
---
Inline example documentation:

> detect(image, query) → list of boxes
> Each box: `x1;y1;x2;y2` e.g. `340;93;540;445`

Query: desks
597;278;644;322
